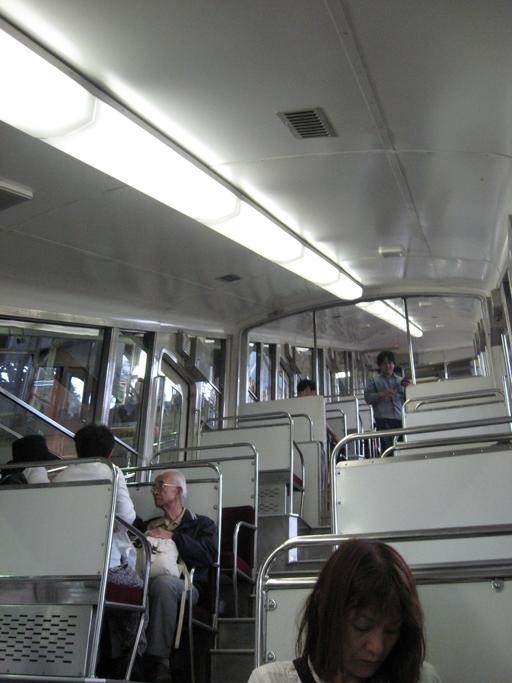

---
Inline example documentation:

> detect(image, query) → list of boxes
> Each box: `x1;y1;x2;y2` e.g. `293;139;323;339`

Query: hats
7;437;62;464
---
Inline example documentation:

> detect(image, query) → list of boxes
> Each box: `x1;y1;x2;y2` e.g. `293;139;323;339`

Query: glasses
151;482;176;491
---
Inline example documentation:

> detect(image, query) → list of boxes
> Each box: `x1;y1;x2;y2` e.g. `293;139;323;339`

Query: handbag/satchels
129;536;180;578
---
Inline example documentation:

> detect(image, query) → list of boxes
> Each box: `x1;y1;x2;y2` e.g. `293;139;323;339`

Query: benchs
330;416;511;563
201;411;306;563
325;394;365;458
240;413;324;525
325;408;348;462
254;527;512;641
359;403;375;433
402;390;510;438
117;463;219;678
148;444;259;616
1;460;152;683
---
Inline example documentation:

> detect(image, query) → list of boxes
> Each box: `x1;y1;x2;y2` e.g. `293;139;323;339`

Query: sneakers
151;663;171;682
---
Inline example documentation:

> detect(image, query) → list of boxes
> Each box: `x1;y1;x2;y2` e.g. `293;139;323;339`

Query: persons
51;423;149;680
246;539;442;683
134;468;217;681
1;434;62;484
296;379;340;444
364;350;411;457
